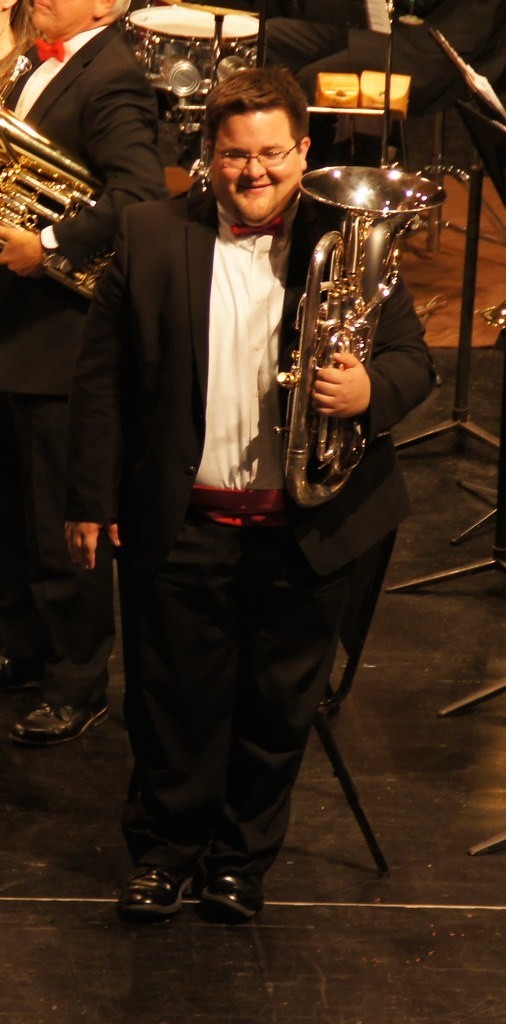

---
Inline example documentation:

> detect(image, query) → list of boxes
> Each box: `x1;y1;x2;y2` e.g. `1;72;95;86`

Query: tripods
381;30;506;595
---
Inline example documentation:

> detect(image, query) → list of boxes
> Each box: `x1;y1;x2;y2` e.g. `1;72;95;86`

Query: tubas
0;103;116;300
276;163;446;508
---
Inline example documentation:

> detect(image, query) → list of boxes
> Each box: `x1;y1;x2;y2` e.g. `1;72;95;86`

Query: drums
125;6;265;120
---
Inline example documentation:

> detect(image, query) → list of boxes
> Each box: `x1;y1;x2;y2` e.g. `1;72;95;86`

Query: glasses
221;144;298;166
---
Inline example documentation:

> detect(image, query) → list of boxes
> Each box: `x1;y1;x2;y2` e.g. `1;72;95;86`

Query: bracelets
40;226;59;254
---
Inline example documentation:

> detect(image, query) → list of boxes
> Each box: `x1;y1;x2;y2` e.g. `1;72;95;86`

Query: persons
0;0;174;745
263;1;504;174
0;0;49;86
59;71;442;928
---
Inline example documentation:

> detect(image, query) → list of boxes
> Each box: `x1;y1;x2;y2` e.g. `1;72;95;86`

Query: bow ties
35;38;64;62
230;218;285;236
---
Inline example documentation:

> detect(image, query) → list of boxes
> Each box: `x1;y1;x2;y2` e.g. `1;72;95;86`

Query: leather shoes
118;866;193;914
8;702;110;745
201;872;264;917
